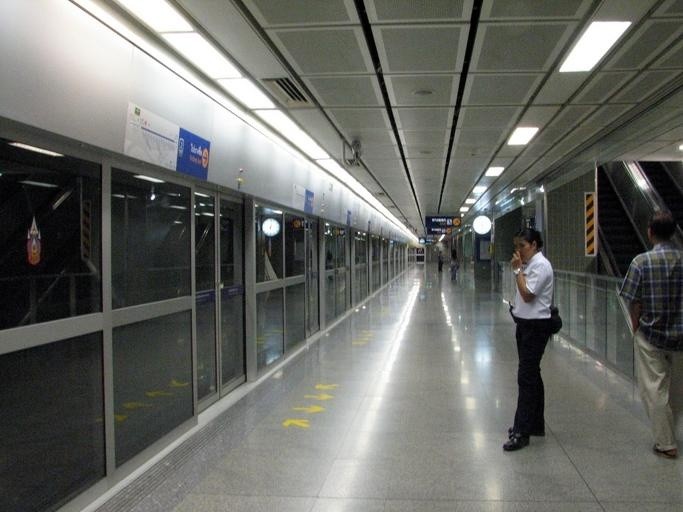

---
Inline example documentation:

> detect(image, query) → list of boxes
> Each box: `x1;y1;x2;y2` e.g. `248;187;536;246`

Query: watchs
512;266;521;276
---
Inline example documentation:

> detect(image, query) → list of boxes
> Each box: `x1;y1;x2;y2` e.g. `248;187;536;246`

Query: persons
500;227;557;452
437;250;444;273
448;248;458;281
617;210;682;459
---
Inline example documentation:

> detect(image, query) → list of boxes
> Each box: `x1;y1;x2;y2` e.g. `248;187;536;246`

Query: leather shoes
502;431;530;451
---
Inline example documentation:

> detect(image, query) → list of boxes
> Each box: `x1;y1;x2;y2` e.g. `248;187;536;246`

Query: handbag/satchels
550;307;563;334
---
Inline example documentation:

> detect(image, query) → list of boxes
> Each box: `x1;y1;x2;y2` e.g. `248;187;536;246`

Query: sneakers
652;442;678;460
